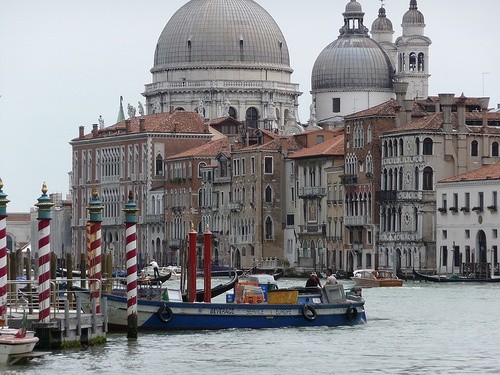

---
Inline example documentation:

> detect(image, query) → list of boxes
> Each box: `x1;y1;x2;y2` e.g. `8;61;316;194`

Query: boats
349;268;404;289
411;269;500;282
116;272;173;287
71;222;369;334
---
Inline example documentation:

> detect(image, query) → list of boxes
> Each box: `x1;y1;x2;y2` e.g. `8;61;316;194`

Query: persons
98;115;105;130
148;259;160;279
152;99;161;114
127;103;136;118
198;98;204;115
267;99;276;119
305;271;322;287
288;100;296;117
144;274;151;280
223;99;231;116
356;272;362;278
137;101;144;116
326;268;338;285
310;102;318;119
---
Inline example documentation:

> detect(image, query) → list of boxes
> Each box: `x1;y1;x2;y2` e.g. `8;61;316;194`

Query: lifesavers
345;306;358;321
156;305;173;324
302;304;318;321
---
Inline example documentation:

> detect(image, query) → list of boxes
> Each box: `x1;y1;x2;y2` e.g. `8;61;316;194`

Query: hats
310;272;318;277
